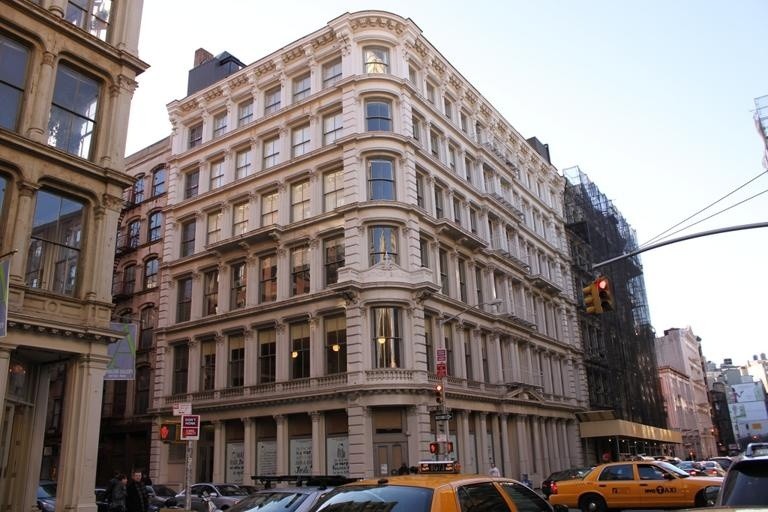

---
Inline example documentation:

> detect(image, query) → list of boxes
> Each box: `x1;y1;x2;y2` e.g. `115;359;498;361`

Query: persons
101;468;149;512
728;388;743;403
488;463;500;477
398;463;410;475
745;424;751;435
520;473;532;489
753;383;763;400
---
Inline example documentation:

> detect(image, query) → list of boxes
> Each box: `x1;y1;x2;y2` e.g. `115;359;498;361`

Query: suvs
714;443;768;509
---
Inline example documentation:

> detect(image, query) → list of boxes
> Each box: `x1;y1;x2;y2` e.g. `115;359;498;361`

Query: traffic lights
158;423;176;444
434;383;443;407
596;274;616;314
580;282;602;316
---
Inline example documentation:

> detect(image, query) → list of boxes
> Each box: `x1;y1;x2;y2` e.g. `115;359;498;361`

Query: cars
541;466;621;498
37;480;58;512
650;455;735;477
174;483;250;512
549;454;727;512
306;460;556;512
146;484;177;512
95;486;110;512
223;474;364;512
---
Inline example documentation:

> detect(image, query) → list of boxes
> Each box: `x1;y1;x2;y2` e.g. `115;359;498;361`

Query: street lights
434;297;505;461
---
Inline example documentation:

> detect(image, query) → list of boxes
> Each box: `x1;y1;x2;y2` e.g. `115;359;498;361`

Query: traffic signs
434;413;453;421
172;402;192;417
179;414;202;441
436;347;448;363
437;363;447;376
